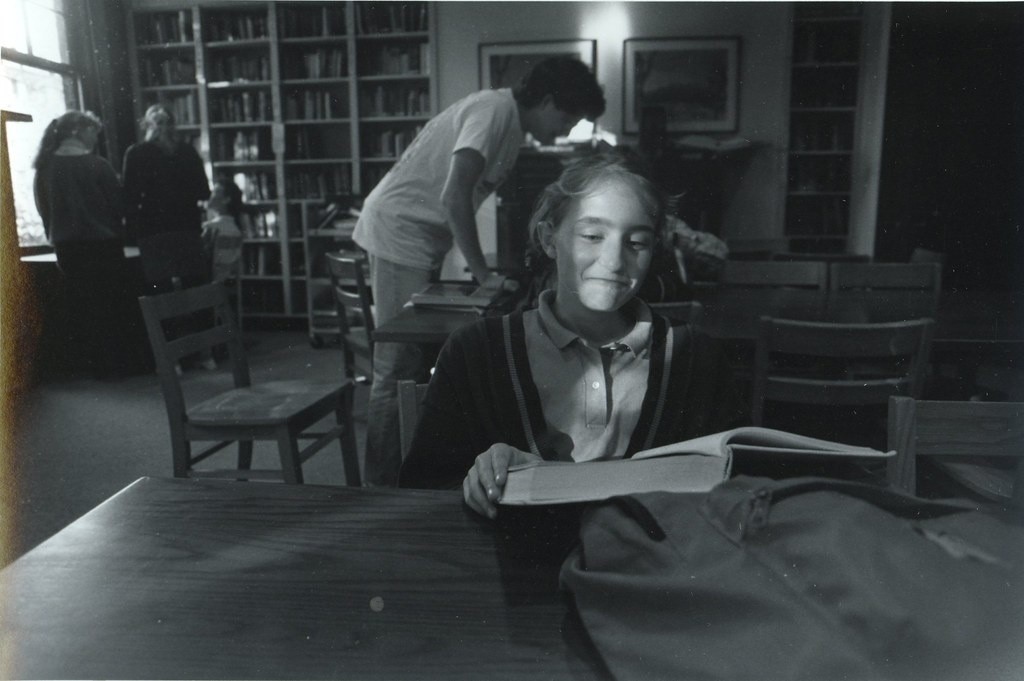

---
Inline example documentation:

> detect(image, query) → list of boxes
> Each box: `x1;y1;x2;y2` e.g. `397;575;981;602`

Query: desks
20;246;138;413
0;474;1024;681
372;279;1024;441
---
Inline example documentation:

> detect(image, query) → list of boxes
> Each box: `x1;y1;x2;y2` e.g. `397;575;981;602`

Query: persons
195;177;250;278
397;154;730;522
30;110;131;377
121;102;221;369
353;53;607;489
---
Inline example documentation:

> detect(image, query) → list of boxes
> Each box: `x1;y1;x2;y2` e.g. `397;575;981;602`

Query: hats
214;178;239;200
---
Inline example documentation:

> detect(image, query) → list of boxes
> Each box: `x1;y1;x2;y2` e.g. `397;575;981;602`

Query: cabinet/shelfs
779;2;865;256
125;0;438;320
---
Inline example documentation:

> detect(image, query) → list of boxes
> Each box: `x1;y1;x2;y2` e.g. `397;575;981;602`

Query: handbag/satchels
559;474;1024;681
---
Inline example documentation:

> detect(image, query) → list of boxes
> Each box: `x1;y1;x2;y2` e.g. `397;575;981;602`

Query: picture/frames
477;38;598;146
622;35;743;137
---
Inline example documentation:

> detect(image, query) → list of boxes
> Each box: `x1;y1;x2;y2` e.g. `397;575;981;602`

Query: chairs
324;253;375;412
717;258;1024;499
137;278;365;489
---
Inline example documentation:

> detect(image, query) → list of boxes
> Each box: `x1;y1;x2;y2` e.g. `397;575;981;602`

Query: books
496;424;899;507
140;2;429;310
